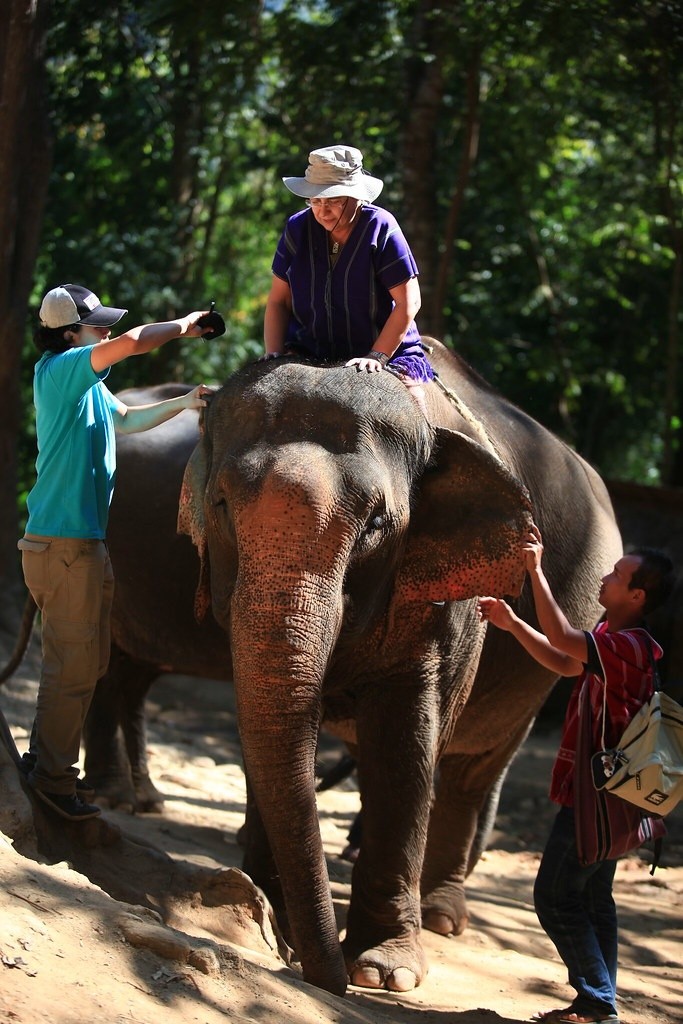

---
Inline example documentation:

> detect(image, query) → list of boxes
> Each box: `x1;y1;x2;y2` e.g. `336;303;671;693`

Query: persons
478;520;677;1023
259;142;437;381
16;282;216;820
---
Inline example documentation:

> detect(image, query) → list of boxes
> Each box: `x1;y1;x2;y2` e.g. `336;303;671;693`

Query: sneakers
35;786;101;821
23;763;95;795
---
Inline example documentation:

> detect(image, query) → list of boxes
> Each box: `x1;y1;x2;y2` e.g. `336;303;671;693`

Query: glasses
304;198;349;211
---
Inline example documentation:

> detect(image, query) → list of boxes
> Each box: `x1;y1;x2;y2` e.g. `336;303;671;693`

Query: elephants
1;336;628;990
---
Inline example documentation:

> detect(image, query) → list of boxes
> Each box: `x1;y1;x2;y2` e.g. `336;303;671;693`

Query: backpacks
593;632;683;817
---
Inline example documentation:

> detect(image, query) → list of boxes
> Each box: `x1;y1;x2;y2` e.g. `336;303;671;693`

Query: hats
38;283;128;328
281;145;383;206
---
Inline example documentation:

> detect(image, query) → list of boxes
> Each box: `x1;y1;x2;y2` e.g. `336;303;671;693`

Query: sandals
533;1003;620;1024
